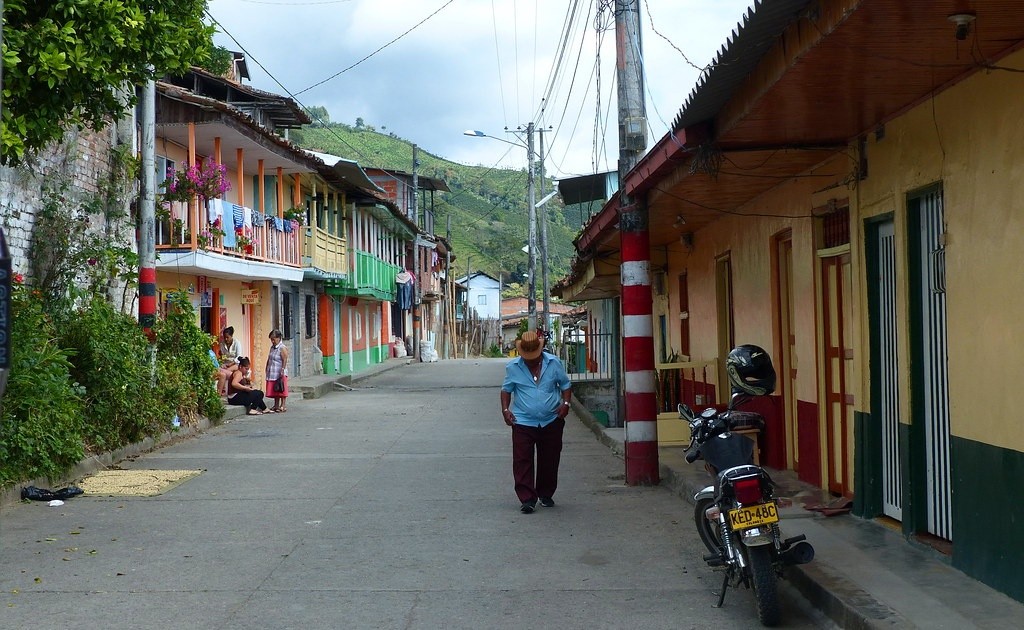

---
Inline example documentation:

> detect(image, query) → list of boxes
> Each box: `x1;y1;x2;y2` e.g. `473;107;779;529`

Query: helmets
727;344;776;395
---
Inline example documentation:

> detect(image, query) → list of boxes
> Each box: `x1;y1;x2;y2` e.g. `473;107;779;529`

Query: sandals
271;406;278;410
276;407;286;412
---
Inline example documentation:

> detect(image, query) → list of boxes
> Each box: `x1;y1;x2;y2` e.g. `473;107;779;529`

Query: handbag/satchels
275;376;284;392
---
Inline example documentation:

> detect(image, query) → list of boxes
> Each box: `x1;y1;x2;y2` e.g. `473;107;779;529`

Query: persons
265;329;288;413
207;326;275;414
501;331;572;513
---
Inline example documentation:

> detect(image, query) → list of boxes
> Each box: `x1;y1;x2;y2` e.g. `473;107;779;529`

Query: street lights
463;130;541;335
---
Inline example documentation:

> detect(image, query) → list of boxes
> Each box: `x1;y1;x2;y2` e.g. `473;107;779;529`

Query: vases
238;243;252;255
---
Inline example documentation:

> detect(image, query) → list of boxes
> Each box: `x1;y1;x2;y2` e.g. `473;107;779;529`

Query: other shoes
520;502;535;512
538;496;553;507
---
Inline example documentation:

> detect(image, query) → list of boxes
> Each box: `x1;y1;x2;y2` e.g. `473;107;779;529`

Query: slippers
263;410;275;413
250;412;263;415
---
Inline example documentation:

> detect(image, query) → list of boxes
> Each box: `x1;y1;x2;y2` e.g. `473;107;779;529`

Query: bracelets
562;401;572;407
502;408;510;414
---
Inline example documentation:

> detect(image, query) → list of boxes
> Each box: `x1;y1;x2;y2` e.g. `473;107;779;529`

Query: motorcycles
678;385;815;627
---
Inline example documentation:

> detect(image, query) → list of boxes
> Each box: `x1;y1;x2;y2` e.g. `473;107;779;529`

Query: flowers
283;202;309;229
158;155;232;254
235;228;259;254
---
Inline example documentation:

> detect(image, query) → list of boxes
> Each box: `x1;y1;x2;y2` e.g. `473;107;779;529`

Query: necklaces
524;360;543;381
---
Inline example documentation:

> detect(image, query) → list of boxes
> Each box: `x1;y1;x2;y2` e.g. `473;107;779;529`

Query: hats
515;332;545;360
